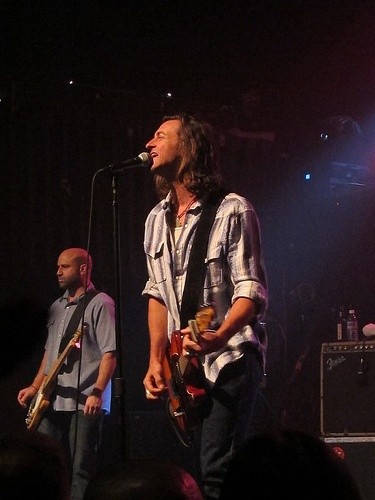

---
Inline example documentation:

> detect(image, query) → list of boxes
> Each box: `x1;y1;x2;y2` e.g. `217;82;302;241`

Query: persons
83;458;204;500
0;432;69;500
219;429;361;500
142;111;270;500
18;248;117;500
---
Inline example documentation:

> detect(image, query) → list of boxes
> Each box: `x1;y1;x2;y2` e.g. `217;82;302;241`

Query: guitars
24;320;90;434
158;298;218;434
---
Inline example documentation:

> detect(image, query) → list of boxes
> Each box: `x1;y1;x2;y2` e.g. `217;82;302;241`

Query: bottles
336;309;346;342
347;310;359;341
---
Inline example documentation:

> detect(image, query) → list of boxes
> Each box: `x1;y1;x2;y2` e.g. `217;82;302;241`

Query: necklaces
172;197;199;223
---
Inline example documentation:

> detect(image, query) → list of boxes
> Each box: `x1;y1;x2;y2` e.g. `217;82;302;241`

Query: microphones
105;151;152;171
358;354;365;378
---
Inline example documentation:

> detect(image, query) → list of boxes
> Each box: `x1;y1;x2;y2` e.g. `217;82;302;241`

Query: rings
145;389;150;394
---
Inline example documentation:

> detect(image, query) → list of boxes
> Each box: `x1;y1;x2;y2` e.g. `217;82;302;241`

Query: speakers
318;350;375;437
323;437;375;500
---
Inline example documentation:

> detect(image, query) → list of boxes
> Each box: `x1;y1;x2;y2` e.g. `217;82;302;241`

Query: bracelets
31;385;38;390
89;388;104;398
